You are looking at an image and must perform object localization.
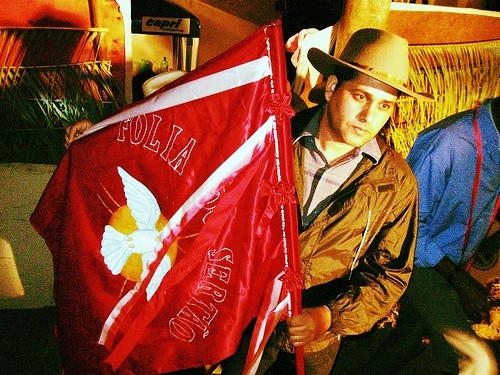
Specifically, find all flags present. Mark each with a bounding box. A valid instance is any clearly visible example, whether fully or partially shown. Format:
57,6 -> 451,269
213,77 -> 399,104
29,19 -> 304,375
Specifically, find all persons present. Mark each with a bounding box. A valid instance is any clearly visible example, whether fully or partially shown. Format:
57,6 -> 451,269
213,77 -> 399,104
65,28 -> 418,375
405,94 -> 500,375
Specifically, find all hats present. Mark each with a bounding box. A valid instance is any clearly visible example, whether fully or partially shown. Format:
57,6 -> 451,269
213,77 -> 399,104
307,28 -> 435,102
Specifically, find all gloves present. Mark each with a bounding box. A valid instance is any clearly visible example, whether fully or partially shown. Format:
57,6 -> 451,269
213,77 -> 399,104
435,256 -> 490,322
